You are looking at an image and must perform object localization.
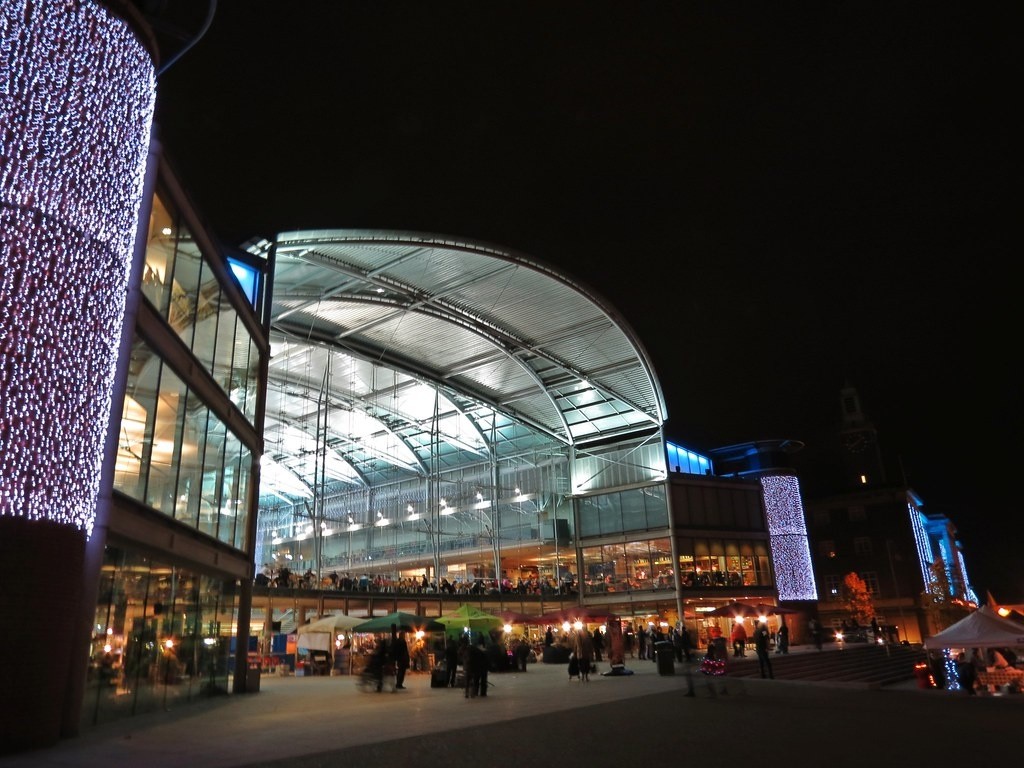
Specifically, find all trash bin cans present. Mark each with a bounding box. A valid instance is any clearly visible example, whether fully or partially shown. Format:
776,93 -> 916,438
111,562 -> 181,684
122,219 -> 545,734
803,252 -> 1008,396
655,641 -> 675,676
246,661 -> 262,693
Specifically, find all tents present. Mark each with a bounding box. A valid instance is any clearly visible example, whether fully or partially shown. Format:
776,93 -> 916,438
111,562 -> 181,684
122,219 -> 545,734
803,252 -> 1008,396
296,602 -> 620,636
925,604 -> 1024,649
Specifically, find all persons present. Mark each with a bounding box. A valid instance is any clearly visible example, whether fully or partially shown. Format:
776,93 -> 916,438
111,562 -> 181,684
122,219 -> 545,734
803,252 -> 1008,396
356,616 -> 860,699
870,616 -> 890,645
259,561 -> 748,598
929,647 -> 1017,697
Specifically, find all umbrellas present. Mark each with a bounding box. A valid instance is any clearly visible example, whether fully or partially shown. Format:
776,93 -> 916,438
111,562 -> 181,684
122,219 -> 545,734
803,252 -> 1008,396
703,603 -> 798,619
